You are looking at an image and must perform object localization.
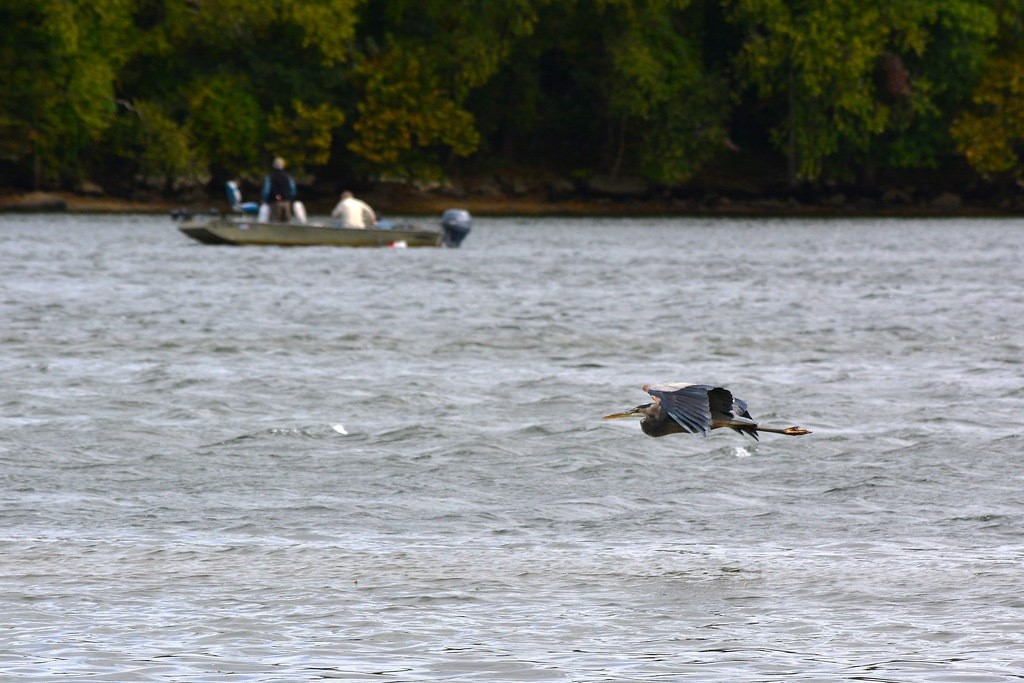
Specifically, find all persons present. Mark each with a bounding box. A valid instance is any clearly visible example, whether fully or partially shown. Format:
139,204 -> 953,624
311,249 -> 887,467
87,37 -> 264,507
261,156 -> 298,225
330,191 -> 377,229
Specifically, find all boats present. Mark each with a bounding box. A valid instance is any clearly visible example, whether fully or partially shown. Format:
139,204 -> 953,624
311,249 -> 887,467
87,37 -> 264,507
179,207 -> 474,249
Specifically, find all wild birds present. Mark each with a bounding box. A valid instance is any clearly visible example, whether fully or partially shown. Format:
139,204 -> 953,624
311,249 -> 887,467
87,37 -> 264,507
600,383 -> 813,442
